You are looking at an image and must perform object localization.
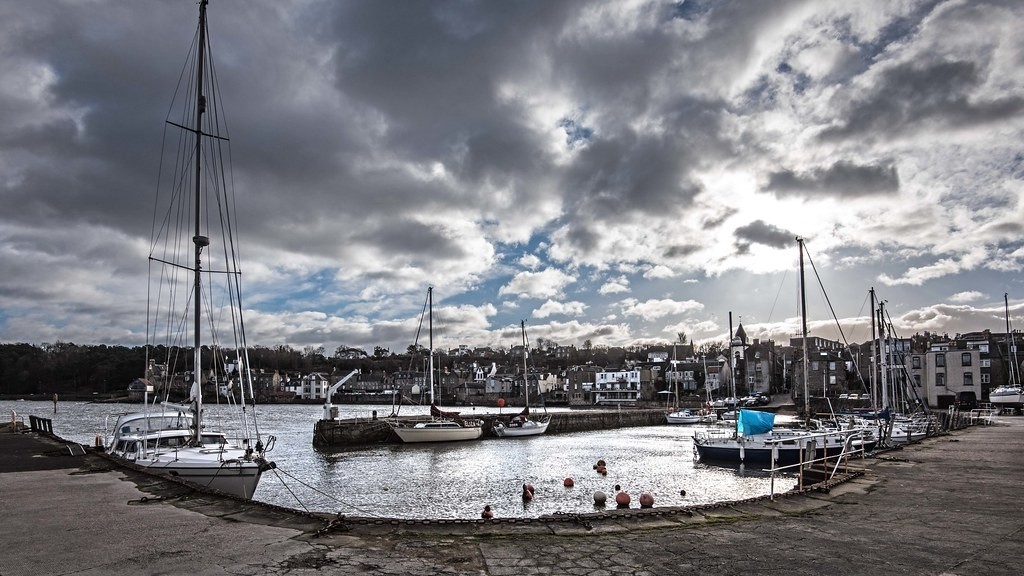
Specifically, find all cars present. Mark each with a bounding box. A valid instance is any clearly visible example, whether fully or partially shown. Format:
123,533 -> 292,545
849,395 -> 858,400
838,393 -> 848,399
860,393 -> 871,400
706,395 -> 771,407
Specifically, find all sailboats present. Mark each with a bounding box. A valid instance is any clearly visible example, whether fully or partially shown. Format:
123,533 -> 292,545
690,234 -> 877,470
989,292 -> 1024,414
492,320 -> 552,438
664,342 -> 701,423
770,287 -> 941,445
108,0 -> 264,501
384,284 -> 484,443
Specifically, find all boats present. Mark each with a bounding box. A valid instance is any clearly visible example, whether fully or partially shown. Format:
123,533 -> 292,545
17,399 -> 25,402
